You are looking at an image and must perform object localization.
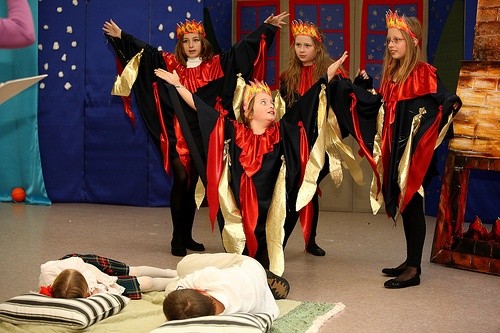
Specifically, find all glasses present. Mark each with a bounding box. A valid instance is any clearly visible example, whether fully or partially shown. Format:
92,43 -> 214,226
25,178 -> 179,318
385,36 -> 404,44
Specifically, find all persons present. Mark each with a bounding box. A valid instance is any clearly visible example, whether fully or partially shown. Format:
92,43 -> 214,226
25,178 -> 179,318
101,10 -> 290,257
153,49 -> 350,276
0,0 -> 35,49
345,8 -> 462,288
278,19 -> 373,257
38,253 -> 176,298
162,253 -> 290,320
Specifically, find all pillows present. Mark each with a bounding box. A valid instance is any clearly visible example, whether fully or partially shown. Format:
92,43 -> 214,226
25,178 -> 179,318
0,294 -> 131,329
149,313 -> 274,333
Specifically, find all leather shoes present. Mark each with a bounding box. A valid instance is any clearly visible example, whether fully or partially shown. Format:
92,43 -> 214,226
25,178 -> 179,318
382,267 -> 421,276
383,278 -> 421,288
190,239 -> 205,252
170,240 -> 188,257
306,245 -> 325,256
